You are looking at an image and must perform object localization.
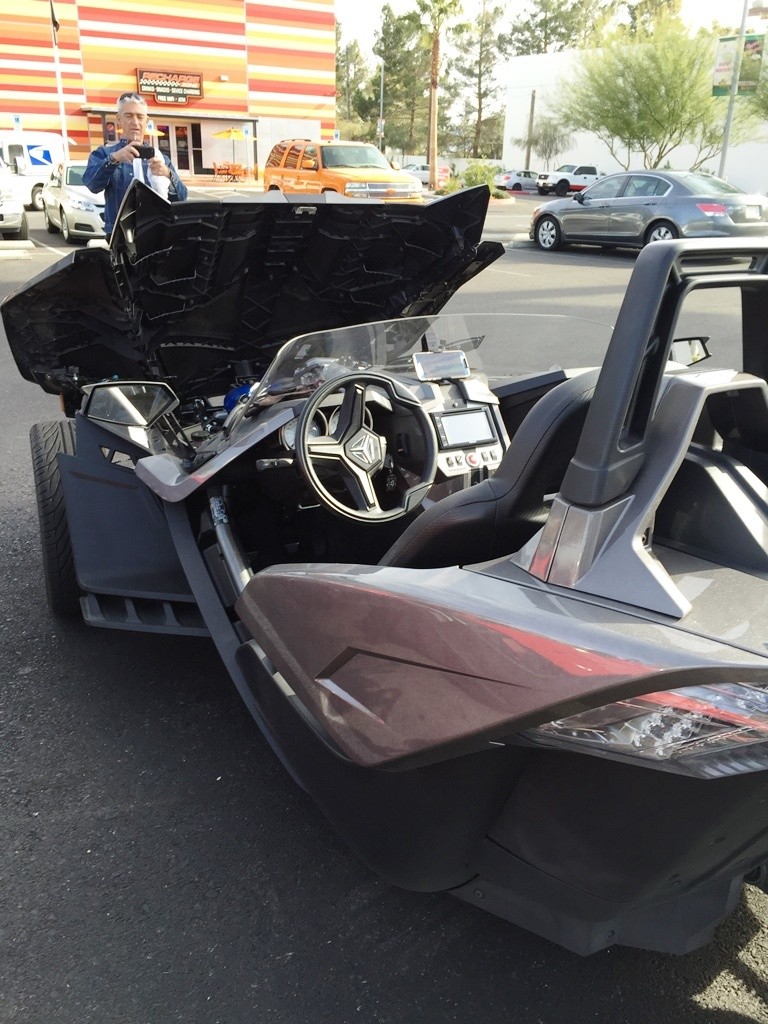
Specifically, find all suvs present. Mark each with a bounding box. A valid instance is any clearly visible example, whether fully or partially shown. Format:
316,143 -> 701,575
535,165 -> 608,196
264,138 -> 425,199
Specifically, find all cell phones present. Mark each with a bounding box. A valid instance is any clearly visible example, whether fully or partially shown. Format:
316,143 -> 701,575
412,350 -> 470,381
132,146 -> 154,158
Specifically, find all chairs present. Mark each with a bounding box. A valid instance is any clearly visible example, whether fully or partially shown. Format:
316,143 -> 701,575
300,368 -> 606,568
69,173 -> 82,184
633,187 -> 648,196
212,160 -> 248,183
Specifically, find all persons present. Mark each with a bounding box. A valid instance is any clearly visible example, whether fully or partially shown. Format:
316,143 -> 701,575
82,92 -> 187,246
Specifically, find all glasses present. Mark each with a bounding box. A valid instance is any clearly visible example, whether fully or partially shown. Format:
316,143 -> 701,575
119,93 -> 144,101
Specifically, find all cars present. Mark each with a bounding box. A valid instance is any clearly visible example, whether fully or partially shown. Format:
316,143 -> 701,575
529,168 -> 768,257
398,164 -> 431,185
493,170 -> 542,190
0,182 -> 28,240
41,158 -> 111,245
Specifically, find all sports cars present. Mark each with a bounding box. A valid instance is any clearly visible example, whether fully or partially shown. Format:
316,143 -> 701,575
1,181 -> 768,961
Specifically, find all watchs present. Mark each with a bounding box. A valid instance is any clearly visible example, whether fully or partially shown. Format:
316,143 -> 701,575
109,152 -> 118,163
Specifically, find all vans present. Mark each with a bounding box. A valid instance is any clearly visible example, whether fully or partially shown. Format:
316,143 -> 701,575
0,129 -> 77,211
